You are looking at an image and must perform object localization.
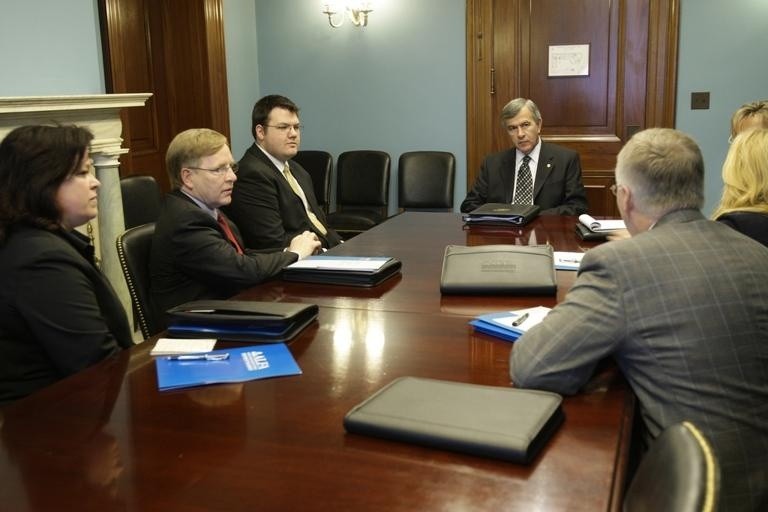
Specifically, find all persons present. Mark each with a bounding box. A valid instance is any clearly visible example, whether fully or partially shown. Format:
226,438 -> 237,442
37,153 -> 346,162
459,97 -> 589,214
232,96 -> 344,253
0,123 -> 134,404
149,129 -> 322,317
710,130 -> 768,248
729,102 -> 768,144
510,128 -> 768,512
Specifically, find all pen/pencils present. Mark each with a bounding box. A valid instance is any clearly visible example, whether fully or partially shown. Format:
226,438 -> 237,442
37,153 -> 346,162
564,259 -> 581,263
165,353 -> 230,361
513,313 -> 528,327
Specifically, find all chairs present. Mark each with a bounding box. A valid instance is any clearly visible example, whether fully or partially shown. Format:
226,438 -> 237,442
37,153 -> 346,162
289,150 -> 333,216
120,174 -> 161,230
115,220 -> 191,341
393,151 -> 455,210
324,150 -> 390,231
614,420 -> 722,511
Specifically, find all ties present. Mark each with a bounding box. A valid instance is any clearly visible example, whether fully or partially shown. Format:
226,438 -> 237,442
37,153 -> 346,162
216,212 -> 244,255
514,157 -> 533,207
283,165 -> 328,237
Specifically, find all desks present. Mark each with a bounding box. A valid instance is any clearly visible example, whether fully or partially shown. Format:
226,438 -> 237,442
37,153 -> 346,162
0,211 -> 640,511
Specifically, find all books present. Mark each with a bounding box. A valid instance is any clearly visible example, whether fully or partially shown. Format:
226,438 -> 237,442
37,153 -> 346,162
343,376 -> 562,462
286,254 -> 393,274
150,337 -> 217,356
283,259 -> 400,289
155,341 -> 305,394
473,304 -> 552,343
574,222 -> 611,245
577,213 -> 626,234
462,202 -> 539,226
167,299 -> 319,345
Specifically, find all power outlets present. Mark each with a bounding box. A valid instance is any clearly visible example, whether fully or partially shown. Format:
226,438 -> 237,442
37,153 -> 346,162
691,92 -> 710,109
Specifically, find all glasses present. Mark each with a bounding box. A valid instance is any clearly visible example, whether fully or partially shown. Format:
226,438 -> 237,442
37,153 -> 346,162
261,123 -> 303,133
183,163 -> 239,176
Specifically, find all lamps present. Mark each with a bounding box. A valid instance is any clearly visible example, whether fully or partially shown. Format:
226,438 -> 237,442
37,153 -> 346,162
320,0 -> 373,28
